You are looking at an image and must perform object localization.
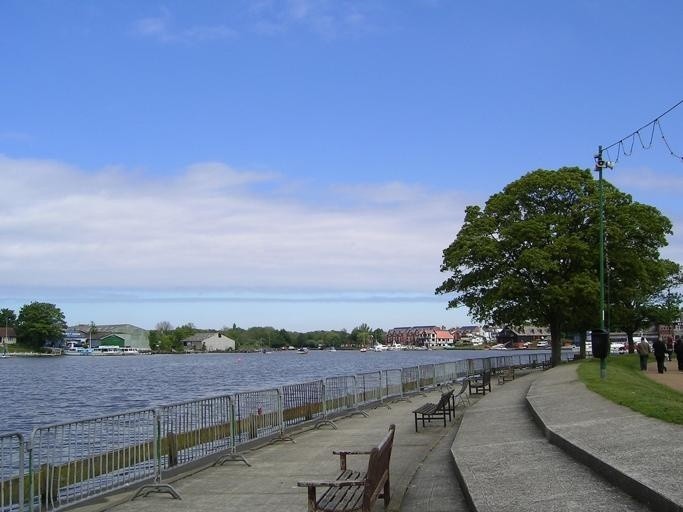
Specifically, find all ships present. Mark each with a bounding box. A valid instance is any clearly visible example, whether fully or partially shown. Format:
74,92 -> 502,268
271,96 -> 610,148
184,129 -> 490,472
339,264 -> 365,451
570,333 -> 652,355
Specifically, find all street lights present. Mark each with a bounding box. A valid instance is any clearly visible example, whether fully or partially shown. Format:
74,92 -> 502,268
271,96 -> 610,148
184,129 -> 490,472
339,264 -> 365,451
593,143 -> 613,377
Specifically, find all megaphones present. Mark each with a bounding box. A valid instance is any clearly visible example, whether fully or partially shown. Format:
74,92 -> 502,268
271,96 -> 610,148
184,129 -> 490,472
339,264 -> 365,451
597,159 -> 606,169
607,159 -> 617,171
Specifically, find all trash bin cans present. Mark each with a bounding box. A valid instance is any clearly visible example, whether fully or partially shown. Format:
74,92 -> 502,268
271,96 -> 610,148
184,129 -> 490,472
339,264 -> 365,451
591,331 -> 609,359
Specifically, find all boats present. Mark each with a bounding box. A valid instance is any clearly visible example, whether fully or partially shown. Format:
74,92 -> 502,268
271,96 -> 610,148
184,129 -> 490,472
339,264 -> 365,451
523,341 -> 532,347
359,347 -> 366,352
536,341 -> 548,347
119,346 -> 139,355
367,339 -> 432,353
90,344 -> 123,356
280,344 -> 336,354
487,343 -> 506,351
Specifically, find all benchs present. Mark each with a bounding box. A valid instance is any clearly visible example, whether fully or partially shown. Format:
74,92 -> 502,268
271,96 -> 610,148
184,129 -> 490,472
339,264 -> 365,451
470,354 -> 580,395
414,389 -> 456,432
296,423 -> 396,512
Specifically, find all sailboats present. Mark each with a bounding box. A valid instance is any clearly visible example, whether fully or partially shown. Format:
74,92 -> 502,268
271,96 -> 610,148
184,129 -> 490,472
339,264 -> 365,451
0,318 -> 15,359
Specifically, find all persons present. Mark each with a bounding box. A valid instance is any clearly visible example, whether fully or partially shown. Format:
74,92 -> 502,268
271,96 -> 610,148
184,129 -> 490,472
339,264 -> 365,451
636,336 -> 650,371
673,334 -> 683,370
651,333 -> 668,374
665,337 -> 674,361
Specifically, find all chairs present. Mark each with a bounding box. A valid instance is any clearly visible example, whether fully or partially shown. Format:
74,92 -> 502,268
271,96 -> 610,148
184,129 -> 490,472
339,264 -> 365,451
454,379 -> 470,406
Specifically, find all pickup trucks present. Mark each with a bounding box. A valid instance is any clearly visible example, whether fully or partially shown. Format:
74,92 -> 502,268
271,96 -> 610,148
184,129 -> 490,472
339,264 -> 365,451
61,341 -> 92,357
